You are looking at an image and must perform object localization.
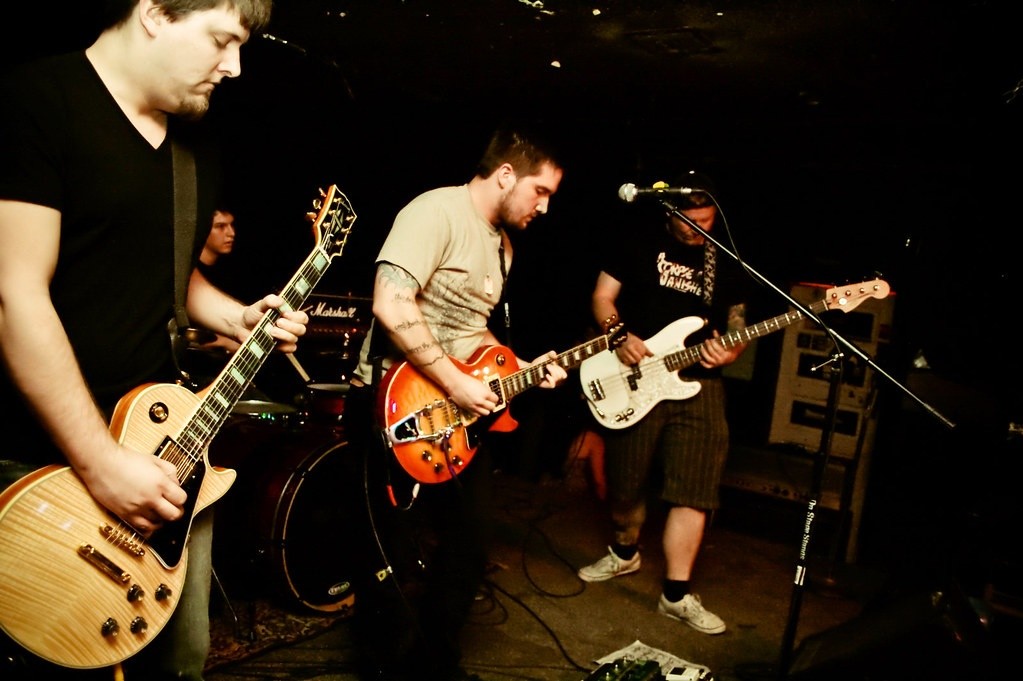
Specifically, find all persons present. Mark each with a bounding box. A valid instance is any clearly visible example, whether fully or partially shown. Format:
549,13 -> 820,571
579,188 -> 747,634
0,0 -> 310,680
352,131 -> 569,681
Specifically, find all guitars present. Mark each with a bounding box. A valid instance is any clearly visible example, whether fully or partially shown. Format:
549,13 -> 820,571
578,270 -> 892,432
373,310 -> 631,487
0,181 -> 357,671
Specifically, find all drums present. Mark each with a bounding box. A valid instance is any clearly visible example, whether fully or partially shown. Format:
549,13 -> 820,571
301,381 -> 352,437
210,430 -> 389,615
218,400 -> 299,450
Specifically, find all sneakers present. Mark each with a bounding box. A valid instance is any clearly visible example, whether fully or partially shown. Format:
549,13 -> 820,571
576,543 -> 643,583
655,591 -> 727,635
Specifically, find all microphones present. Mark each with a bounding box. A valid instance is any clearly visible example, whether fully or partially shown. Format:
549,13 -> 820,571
618,182 -> 692,202
